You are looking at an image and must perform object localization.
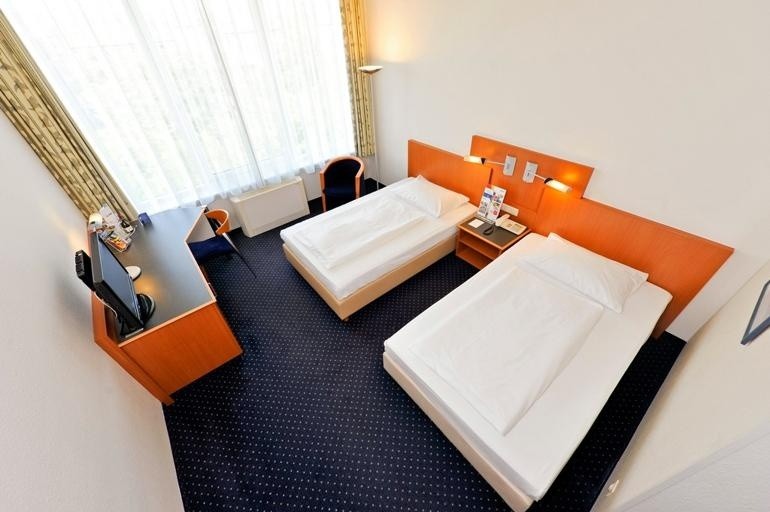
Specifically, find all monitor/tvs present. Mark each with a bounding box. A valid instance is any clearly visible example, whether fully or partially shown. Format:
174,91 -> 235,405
89,231 -> 155,338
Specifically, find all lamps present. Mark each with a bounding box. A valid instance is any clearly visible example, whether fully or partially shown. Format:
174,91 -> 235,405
464,154 -> 517,176
359,66 -> 384,190
521,160 -> 573,194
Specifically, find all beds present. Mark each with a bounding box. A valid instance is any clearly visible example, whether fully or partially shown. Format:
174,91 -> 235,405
381,235 -> 673,512
280,176 -> 480,321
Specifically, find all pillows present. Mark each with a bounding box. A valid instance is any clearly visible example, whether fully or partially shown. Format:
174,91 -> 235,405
390,173 -> 471,218
525,231 -> 649,315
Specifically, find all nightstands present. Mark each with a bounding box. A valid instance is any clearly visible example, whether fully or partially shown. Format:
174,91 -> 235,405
456,210 -> 533,270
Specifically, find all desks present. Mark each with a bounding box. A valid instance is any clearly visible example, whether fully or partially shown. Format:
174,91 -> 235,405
87,205 -> 244,407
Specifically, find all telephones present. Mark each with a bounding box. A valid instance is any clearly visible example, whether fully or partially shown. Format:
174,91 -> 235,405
495,214 -> 527,236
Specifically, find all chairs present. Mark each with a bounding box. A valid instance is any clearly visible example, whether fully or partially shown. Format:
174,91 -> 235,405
187,209 -> 257,279
320,156 -> 365,212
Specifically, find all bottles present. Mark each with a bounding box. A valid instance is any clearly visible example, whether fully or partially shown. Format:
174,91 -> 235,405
121,219 -> 133,234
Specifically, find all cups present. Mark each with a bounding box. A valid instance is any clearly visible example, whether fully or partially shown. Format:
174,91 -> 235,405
138,212 -> 151,226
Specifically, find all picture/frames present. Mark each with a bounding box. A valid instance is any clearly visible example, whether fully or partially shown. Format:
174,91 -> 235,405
740,279 -> 770,345
